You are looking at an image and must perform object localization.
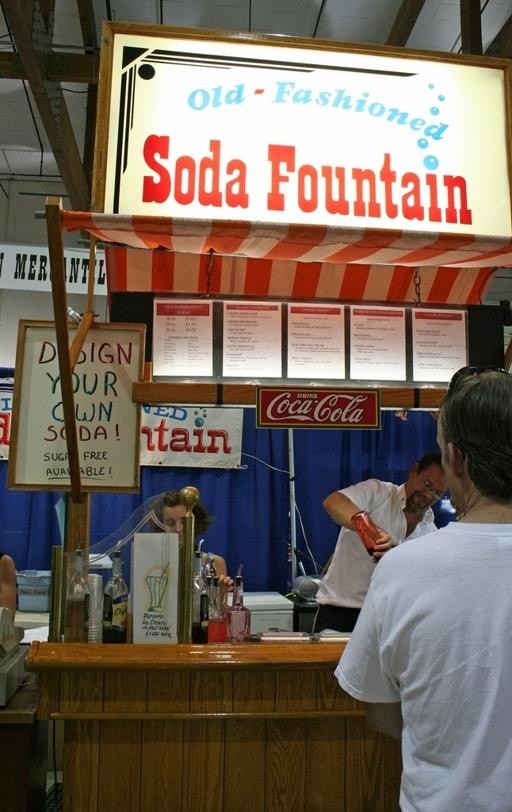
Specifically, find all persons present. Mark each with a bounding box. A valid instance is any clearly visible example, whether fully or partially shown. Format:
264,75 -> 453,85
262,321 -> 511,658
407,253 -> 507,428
310,450 -> 447,634
334,367 -> 512,812
155,489 -> 234,607
0,551 -> 18,626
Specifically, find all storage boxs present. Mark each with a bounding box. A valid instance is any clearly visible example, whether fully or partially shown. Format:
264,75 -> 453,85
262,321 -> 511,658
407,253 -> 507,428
15,570 -> 52,612
88,553 -> 113,588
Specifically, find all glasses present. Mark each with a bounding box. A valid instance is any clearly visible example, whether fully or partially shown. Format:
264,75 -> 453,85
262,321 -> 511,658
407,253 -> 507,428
447,366 -> 509,391
420,467 -> 447,502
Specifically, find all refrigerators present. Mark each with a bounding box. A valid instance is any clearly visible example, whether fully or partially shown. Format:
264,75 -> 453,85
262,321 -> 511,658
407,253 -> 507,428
224,589 -> 295,637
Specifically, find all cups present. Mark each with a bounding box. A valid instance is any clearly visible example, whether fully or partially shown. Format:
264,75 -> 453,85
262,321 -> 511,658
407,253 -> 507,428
85,571 -> 105,644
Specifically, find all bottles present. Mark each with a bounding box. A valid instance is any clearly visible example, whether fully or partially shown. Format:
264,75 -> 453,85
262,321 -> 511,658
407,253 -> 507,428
350,511 -> 384,564
102,550 -> 130,645
190,548 -> 254,646
64,548 -> 89,644
